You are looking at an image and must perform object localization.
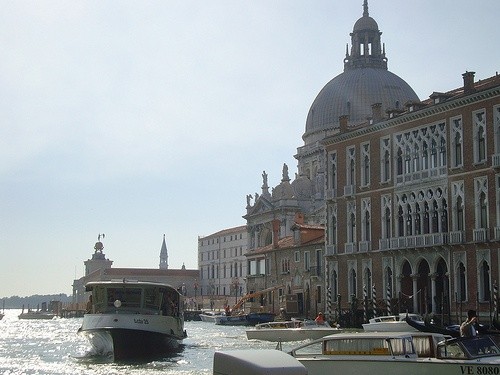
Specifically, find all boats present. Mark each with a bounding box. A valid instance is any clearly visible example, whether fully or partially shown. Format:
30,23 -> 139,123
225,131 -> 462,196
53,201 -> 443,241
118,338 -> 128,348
18,313 -> 57,319
199,312 -> 276,325
287,331 -> 500,375
246,322 -> 343,342
77,280 -> 188,363
361,316 -> 434,332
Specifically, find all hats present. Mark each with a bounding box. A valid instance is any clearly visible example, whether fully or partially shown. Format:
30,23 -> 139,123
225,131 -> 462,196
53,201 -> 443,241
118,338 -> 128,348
468,310 -> 478,317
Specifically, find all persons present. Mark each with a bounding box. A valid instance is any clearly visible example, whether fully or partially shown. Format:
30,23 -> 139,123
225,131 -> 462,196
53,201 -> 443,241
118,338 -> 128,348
86,295 -> 92,310
460,310 -> 483,337
163,298 -> 170,315
226,305 -> 230,316
316,312 -> 323,325
